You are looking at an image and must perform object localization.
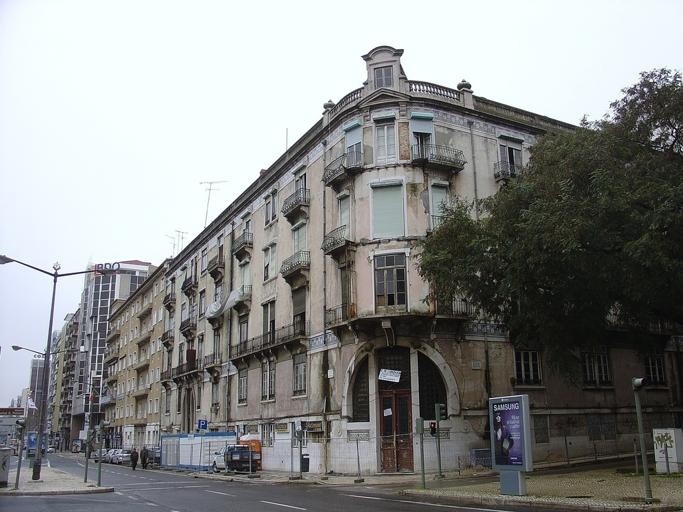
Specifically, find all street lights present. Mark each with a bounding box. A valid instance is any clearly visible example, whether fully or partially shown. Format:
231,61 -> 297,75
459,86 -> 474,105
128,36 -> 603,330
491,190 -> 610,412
10,343 -> 79,468
0,251 -> 116,485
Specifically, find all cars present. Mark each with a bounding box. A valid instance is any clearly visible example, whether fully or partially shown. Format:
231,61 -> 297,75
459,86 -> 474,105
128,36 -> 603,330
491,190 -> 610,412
21,430 -> 160,472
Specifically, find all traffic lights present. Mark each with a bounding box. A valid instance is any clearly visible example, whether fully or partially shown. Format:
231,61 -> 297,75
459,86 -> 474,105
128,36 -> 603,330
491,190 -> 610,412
434,403 -> 446,423
16,418 -> 25,427
429,421 -> 436,437
631,377 -> 648,390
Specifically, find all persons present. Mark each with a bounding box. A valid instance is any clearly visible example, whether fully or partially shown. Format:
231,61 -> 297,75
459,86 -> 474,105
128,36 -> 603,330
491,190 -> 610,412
129,448 -> 138,470
140,446 -> 148,468
494,412 -> 514,464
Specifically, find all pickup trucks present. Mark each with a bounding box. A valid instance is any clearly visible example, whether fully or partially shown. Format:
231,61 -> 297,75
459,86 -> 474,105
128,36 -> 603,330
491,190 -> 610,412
210,443 -> 261,475
